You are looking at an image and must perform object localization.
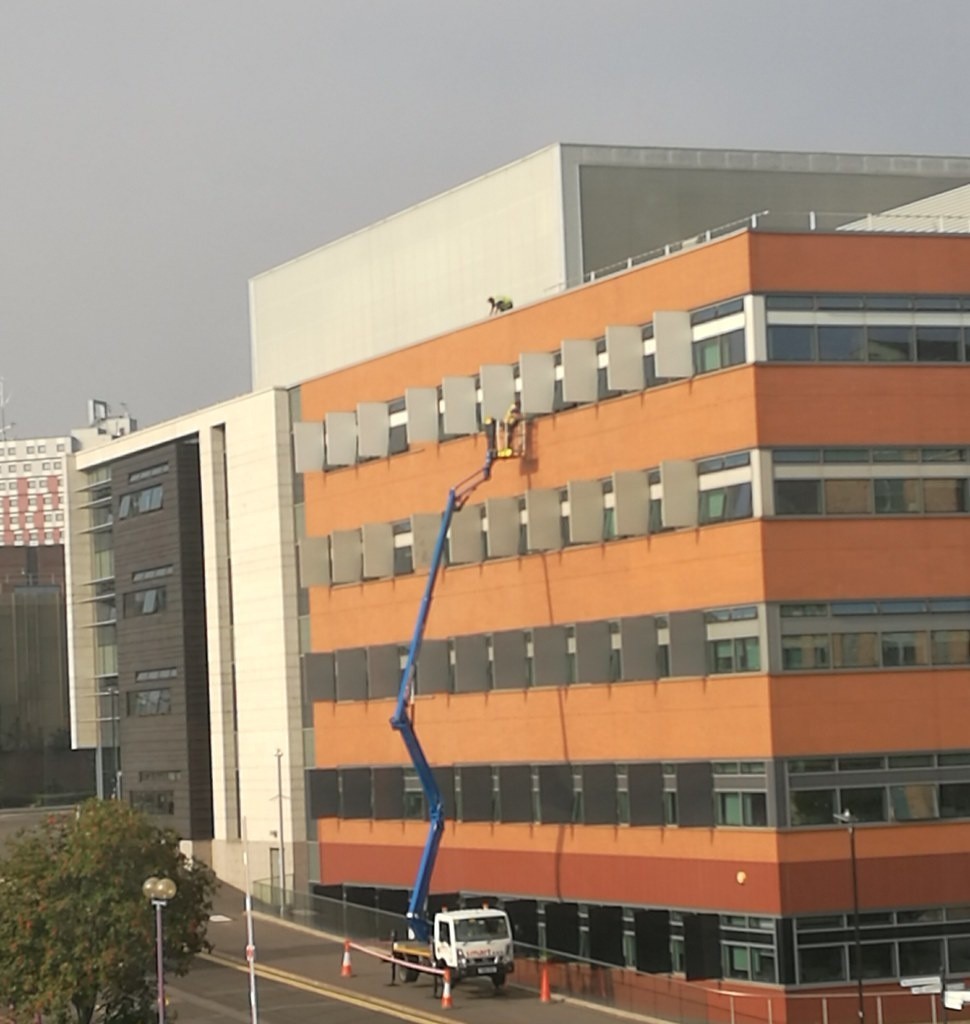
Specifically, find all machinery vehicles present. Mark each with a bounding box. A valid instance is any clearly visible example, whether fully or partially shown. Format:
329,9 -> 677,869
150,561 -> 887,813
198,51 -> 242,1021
387,406 -> 523,992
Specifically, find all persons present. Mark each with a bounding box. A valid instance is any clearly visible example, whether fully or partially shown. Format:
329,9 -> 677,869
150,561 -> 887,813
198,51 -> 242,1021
489,296 -> 514,316
501,400 -> 523,455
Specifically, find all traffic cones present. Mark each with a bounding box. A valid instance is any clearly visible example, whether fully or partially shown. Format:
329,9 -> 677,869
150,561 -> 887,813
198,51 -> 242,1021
339,939 -> 354,981
540,969 -> 550,1004
437,966 -> 457,1011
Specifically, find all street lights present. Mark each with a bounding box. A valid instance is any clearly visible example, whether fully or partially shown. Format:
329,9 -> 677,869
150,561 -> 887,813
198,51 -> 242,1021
106,684 -> 118,802
141,875 -> 179,1024
272,745 -> 289,919
834,806 -> 865,1023
